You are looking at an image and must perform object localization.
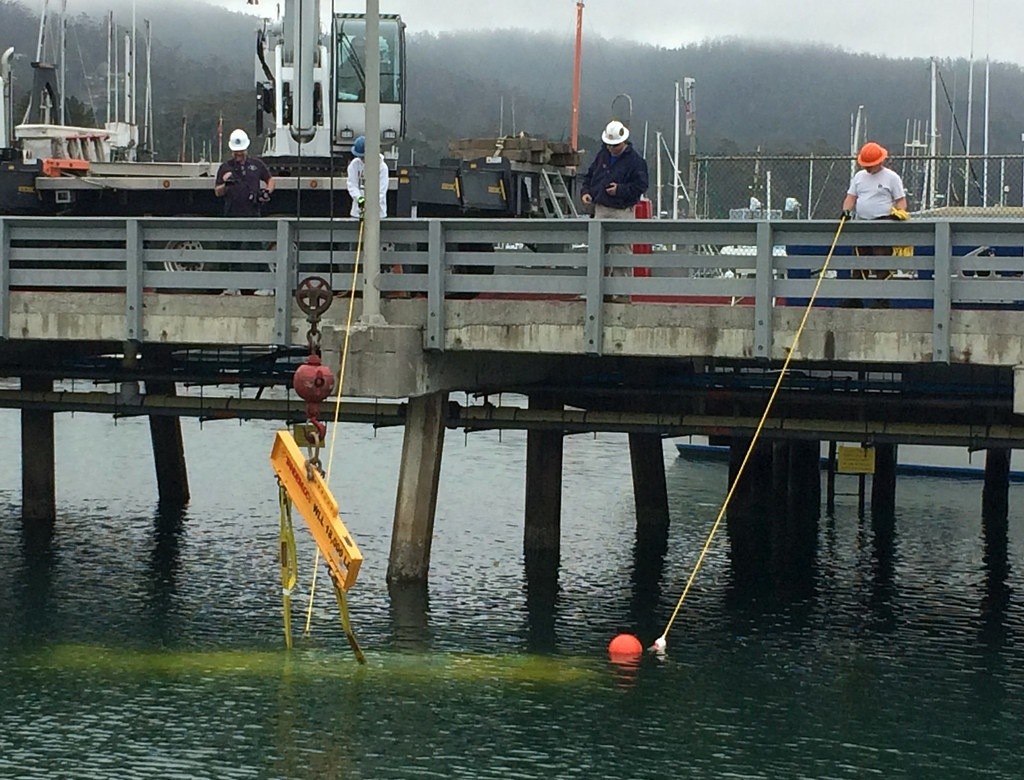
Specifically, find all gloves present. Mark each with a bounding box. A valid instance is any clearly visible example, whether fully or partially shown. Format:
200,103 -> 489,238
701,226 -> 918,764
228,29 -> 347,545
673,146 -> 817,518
357,196 -> 367,209
840,210 -> 852,221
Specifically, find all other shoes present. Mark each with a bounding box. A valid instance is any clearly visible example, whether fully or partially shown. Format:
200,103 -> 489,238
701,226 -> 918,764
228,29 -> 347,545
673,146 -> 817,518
609,294 -> 632,304
870,298 -> 891,308
843,298 -> 864,308
577,294 -> 586,301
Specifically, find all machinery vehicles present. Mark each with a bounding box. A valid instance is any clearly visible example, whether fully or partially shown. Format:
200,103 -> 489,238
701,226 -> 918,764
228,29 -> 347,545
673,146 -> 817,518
0,0 -> 587,593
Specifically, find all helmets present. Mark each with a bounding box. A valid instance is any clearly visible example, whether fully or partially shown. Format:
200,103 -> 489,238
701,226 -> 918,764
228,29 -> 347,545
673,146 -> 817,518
351,135 -> 366,156
858,142 -> 888,166
601,120 -> 630,144
228,129 -> 250,151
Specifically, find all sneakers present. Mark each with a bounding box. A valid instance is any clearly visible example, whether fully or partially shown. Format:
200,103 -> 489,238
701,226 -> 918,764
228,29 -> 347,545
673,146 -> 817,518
254,288 -> 275,296
222,288 -> 242,296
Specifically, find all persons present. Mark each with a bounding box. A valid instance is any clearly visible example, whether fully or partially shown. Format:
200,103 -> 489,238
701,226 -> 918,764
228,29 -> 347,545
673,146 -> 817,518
335,35 -> 367,97
215,129 -> 276,297
346,136 -> 389,297
839,142 -> 907,309
565,120 -> 649,306
